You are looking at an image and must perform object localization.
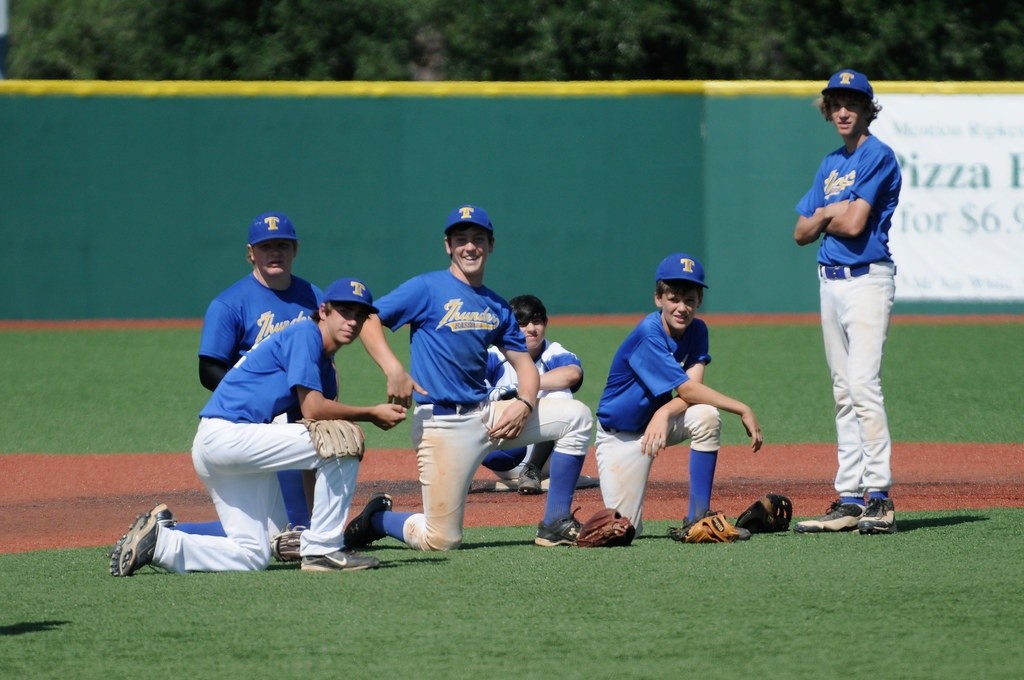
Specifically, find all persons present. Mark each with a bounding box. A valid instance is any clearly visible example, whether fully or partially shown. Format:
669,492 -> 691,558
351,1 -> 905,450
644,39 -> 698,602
794,70 -> 902,535
468,295 -> 600,496
595,253 -> 763,540
342,205 -> 594,551
110,278 -> 408,571
149,214 -> 339,538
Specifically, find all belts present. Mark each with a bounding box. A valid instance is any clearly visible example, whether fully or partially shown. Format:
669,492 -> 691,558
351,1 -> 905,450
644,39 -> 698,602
601,423 -> 620,433
820,263 -> 869,279
433,402 -> 480,416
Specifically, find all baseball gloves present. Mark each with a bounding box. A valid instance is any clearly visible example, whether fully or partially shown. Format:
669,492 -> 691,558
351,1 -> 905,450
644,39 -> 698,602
294,415 -> 367,462
576,508 -> 636,547
684,514 -> 739,544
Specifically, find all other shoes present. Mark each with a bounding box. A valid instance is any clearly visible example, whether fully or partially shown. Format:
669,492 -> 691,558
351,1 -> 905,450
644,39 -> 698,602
737,527 -> 752,540
148,503 -> 177,528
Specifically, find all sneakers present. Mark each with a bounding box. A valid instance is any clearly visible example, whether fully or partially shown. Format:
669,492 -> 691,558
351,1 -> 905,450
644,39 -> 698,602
534,506 -> 584,546
857,496 -> 897,534
793,499 -> 867,534
517,461 -> 542,495
301,551 -> 381,572
109,513 -> 162,577
343,493 -> 392,550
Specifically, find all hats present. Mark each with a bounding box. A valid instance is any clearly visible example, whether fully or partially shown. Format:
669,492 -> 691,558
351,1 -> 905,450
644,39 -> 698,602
249,212 -> 302,245
821,69 -> 874,100
655,253 -> 709,289
321,278 -> 380,314
444,204 -> 493,234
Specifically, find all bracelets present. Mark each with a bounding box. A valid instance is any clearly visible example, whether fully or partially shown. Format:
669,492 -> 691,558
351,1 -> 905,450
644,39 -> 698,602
518,398 -> 533,412
